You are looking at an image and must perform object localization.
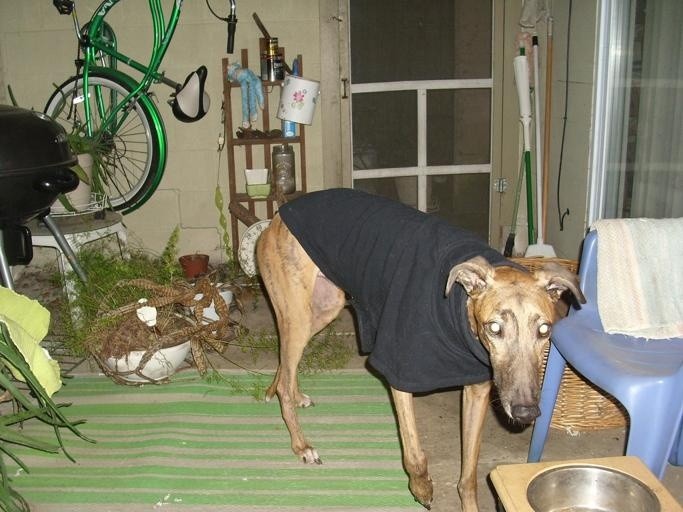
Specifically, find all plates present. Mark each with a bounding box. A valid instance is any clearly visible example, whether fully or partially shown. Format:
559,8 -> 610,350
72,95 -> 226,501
238,220 -> 273,279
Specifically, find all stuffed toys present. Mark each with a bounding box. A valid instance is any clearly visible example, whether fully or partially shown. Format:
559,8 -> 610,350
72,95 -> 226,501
227,62 -> 264,128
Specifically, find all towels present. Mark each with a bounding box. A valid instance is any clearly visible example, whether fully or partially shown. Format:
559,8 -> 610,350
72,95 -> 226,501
590,217 -> 683,340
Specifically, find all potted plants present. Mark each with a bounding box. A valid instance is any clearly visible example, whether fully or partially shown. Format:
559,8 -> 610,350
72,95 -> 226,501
52,226 -> 354,403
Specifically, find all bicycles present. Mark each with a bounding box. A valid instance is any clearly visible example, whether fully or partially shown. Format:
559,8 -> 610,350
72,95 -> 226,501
43,0 -> 238,216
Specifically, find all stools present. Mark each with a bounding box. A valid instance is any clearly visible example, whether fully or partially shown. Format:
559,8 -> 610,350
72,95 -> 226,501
8,212 -> 132,338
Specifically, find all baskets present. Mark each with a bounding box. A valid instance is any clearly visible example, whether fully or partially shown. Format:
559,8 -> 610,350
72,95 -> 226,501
511,258 -> 632,434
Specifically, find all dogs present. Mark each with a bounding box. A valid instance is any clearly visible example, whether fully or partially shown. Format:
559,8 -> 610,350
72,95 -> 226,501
256,188 -> 586,512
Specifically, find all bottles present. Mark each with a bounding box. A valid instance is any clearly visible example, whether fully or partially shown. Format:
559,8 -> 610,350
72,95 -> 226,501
273,144 -> 295,195
281,121 -> 295,136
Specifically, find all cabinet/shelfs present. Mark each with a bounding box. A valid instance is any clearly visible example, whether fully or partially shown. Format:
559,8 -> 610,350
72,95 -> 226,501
221,49 -> 307,277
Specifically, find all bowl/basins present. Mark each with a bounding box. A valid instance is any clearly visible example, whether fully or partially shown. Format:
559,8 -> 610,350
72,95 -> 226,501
526,466 -> 660,512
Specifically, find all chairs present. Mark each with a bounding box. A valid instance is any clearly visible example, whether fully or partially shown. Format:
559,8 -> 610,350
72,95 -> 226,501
525,218 -> 683,481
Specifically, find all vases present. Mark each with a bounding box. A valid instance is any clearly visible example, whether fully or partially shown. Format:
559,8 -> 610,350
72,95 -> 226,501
178,254 -> 209,279
182,283 -> 233,337
